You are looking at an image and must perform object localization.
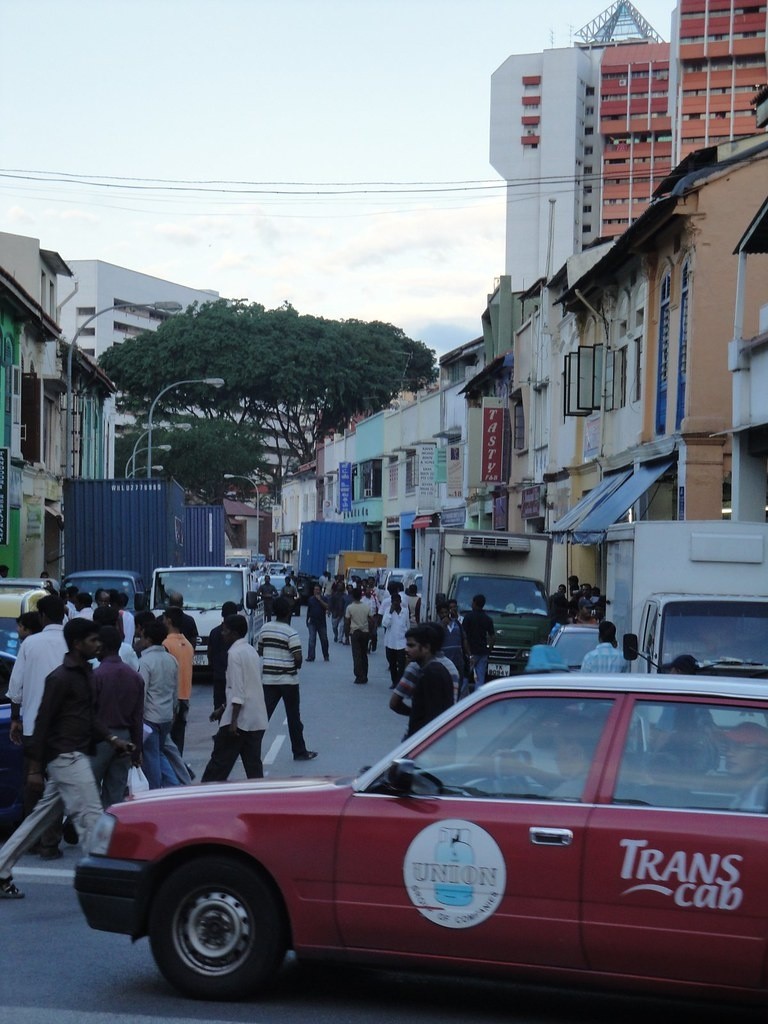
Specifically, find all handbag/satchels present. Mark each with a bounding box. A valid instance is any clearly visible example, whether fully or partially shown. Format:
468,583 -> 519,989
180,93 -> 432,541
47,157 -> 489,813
124,765 -> 150,796
463,656 -> 476,683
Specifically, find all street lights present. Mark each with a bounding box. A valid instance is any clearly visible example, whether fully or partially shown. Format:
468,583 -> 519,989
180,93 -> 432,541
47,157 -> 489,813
66,299 -> 183,478
224,474 -> 259,554
148,378 -> 226,477
132,423 -> 192,476
125,445 -> 171,478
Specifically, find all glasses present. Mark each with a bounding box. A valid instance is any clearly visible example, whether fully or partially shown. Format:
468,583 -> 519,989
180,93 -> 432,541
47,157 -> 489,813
725,743 -> 763,752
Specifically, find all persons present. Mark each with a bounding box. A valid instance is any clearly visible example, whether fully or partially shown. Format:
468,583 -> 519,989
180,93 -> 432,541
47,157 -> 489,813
481,725 -> 598,801
305,583 -> 330,663
345,588 -> 372,686
0,618 -> 138,899
654,655 -> 713,726
200,613 -> 269,783
0,562 -> 633,855
257,596 -> 318,761
713,722 -> 768,811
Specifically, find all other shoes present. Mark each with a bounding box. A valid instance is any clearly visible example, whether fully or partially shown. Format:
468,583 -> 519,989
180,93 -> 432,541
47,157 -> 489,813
324,655 -> 329,661
294,750 -> 318,760
0,875 -> 25,899
305,658 -> 314,662
62,818 -> 79,845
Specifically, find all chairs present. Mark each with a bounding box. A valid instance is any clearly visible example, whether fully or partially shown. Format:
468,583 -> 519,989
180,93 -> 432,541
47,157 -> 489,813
620,742 -> 689,806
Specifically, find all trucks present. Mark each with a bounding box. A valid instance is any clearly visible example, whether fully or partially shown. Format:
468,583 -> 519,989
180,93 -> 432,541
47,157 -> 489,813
605,520 -> 768,680
337,550 -> 388,590
418,526 -> 553,681
148,565 -> 267,676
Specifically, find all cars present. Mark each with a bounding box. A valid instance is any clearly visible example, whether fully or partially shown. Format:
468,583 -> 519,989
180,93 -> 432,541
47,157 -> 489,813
0,650 -> 52,832
74,673 -> 768,1024
225,557 -> 259,568
260,562 -> 294,575
259,575 -> 300,615
550,623 -> 604,673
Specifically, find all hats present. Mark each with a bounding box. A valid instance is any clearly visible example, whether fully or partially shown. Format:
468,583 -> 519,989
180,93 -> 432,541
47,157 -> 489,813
721,721 -> 768,748
579,599 -> 593,607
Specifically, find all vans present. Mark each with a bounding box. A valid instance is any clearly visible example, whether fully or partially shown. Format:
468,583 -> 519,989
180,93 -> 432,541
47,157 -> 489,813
373,568 -> 414,605
0,576 -> 61,595
0,586 -> 52,659
59,569 -> 147,616
403,571 -> 423,599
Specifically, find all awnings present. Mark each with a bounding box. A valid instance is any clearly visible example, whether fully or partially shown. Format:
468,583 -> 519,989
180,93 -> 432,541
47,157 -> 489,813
547,463 -> 676,540
224,497 -> 273,519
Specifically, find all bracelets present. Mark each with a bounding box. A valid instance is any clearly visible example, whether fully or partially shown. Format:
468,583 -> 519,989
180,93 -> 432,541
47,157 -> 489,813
222,703 -> 226,709
109,736 -> 118,742
